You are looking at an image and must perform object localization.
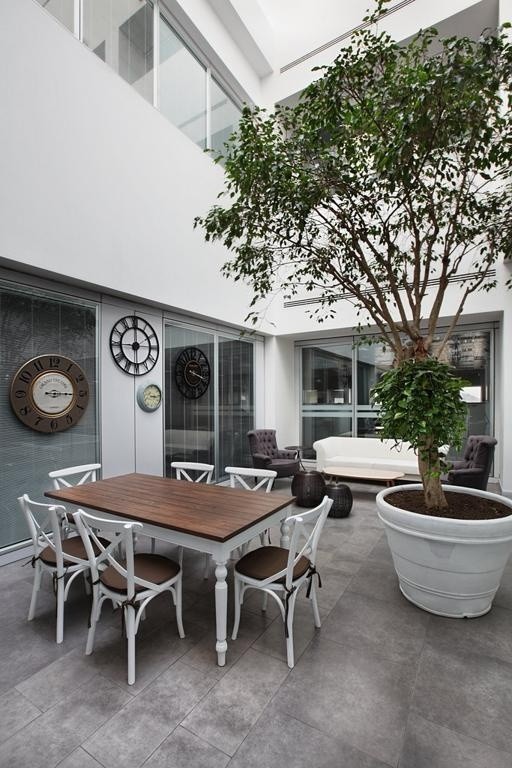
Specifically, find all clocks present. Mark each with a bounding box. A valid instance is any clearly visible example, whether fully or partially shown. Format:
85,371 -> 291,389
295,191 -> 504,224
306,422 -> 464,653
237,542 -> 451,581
10,315 -> 211,436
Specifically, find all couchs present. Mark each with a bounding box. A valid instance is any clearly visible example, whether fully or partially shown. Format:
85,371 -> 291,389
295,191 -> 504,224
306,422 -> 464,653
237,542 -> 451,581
312,436 -> 450,482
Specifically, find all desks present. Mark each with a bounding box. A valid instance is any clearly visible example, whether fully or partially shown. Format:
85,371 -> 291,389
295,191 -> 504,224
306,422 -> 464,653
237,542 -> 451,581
283,446 -> 313,471
43,473 -> 297,667
322,466 -> 406,488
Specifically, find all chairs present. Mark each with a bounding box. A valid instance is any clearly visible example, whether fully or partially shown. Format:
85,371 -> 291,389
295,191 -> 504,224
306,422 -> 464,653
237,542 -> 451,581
17,493 -> 119,644
47,460 -> 122,540
246,429 -> 300,485
445,435 -> 498,491
72,507 -> 187,685
152,461 -> 215,573
204,466 -> 280,578
231,495 -> 335,669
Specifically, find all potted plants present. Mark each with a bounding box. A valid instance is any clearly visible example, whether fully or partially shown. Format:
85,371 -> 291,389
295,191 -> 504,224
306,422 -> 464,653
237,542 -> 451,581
193,0 -> 512,618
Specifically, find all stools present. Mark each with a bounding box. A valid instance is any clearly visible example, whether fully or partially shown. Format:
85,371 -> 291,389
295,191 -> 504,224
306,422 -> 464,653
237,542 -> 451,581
322,484 -> 354,519
290,470 -> 326,507
